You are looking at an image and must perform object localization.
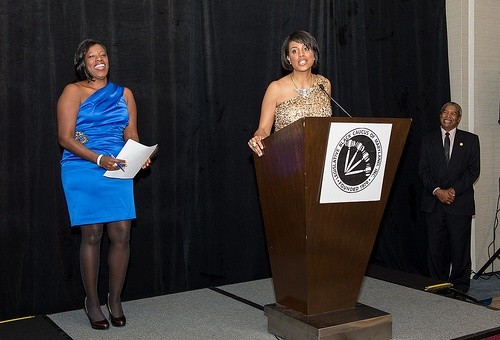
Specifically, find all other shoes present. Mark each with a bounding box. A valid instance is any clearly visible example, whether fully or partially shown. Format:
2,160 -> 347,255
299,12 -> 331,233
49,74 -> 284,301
453,291 -> 466,301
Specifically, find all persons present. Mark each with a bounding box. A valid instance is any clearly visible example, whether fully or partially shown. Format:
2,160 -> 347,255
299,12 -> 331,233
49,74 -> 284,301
57,39 -> 151,330
248,31 -> 332,157
417,102 -> 480,300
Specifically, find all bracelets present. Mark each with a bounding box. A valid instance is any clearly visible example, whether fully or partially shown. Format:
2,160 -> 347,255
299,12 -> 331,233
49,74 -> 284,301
96,154 -> 103,166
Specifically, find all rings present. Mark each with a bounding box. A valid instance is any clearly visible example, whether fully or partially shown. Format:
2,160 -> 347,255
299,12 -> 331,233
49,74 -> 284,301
253,142 -> 256,147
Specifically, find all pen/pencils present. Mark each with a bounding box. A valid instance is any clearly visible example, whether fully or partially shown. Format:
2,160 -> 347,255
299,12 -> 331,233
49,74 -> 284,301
106,151 -> 126,173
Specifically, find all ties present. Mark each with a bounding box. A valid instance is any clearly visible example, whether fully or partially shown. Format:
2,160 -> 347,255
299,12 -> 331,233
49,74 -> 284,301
442,131 -> 451,160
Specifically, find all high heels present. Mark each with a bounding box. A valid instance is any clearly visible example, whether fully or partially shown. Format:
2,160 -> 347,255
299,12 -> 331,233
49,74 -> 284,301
102,296 -> 127,329
82,294 -> 109,333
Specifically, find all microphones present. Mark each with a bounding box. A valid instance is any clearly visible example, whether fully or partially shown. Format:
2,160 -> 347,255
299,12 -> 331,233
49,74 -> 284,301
319,84 -> 352,117
